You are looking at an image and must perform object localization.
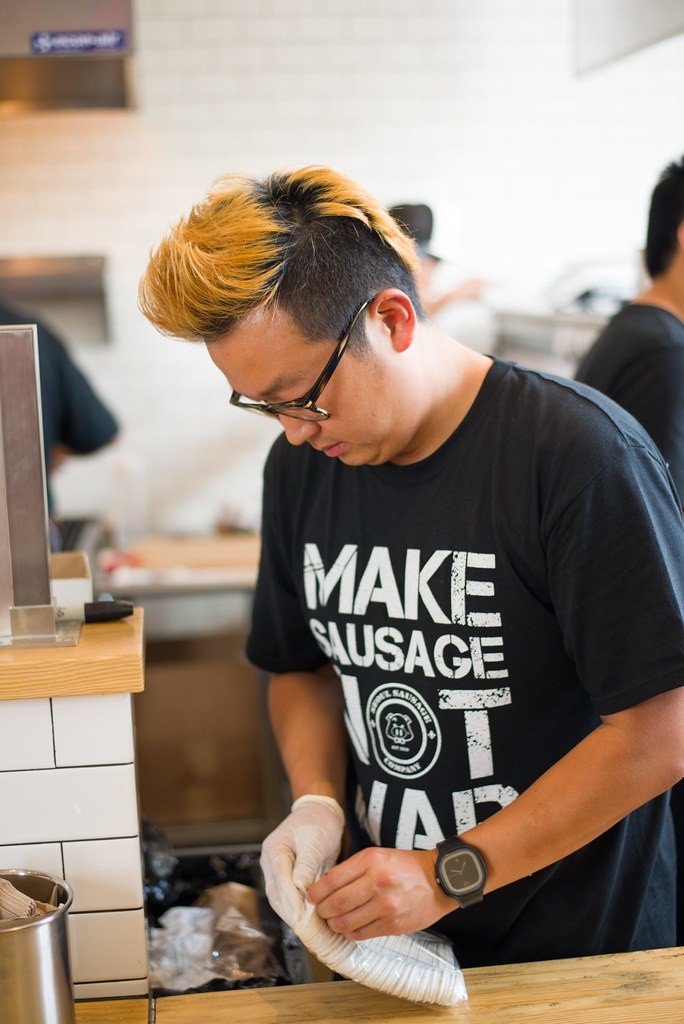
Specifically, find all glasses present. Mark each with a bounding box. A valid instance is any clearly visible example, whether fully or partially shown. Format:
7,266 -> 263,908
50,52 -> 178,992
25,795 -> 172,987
229,294 -> 376,420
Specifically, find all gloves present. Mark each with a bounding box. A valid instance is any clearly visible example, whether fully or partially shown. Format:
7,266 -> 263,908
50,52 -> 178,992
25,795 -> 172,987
261,795 -> 346,928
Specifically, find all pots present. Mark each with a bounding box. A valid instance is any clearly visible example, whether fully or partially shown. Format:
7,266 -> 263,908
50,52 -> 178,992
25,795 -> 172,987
0,867 -> 76,1024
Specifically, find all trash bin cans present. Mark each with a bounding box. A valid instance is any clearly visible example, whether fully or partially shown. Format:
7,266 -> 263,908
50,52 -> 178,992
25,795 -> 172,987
147,841 -> 316,997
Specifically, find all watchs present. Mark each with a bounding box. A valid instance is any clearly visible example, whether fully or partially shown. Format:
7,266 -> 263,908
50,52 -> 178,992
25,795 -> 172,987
435,835 -> 486,910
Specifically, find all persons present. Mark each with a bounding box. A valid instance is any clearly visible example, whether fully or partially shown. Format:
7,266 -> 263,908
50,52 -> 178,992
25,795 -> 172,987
576,156 -> 684,511
386,203 -> 498,355
0,295 -> 117,552
141,166 -> 684,970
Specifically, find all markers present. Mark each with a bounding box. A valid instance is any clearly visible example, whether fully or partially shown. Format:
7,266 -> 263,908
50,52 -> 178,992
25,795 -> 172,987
55,600 -> 136,629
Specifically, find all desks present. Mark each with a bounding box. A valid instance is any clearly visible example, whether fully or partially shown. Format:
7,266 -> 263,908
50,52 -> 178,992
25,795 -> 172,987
72,946 -> 684,1024
0,604 -> 145,700
